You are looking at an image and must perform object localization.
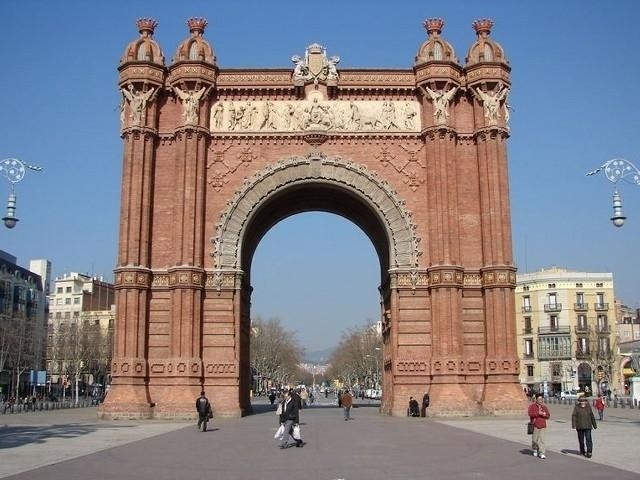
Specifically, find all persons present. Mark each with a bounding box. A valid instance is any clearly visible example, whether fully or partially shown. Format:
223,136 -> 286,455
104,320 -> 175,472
196,391 -> 209,432
595,394 -> 605,420
340,390 -> 352,421
337,392 -> 342,407
421,392 -> 429,417
213,101 -> 417,131
572,395 -> 597,458
409,397 -> 420,417
324,389 -> 328,398
528,393 -> 550,458
269,385 -> 314,448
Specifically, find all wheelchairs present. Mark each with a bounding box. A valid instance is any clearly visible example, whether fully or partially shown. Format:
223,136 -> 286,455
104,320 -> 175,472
410,406 -> 420,417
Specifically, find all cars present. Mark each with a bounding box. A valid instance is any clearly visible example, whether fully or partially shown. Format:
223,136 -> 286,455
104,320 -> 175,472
364,389 -> 382,399
561,389 -> 585,400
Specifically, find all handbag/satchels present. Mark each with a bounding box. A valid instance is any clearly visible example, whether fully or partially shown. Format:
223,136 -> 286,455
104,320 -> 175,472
276,403 -> 283,415
527,423 -> 534,434
293,424 -> 301,440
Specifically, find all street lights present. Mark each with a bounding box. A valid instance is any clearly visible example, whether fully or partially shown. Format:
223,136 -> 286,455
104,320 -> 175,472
366,355 -> 379,389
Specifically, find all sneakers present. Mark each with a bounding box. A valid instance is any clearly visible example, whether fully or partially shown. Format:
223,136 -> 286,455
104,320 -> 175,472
533,450 -> 538,456
539,453 -> 546,459
587,453 -> 592,457
